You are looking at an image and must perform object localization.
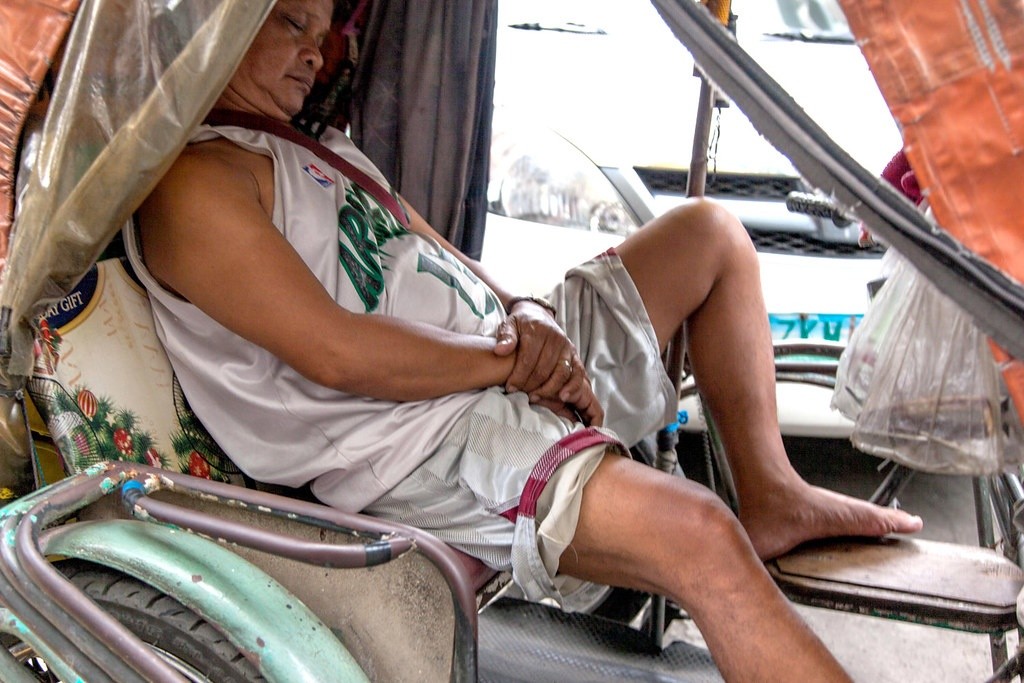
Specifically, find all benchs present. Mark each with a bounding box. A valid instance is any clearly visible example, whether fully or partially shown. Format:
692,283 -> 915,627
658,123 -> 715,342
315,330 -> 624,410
30,251 -> 516,614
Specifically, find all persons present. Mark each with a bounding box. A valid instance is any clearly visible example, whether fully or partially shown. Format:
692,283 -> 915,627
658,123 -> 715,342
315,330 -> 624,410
121,1 -> 923,683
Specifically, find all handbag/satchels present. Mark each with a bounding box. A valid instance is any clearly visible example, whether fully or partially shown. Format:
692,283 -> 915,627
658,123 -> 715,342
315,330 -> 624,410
830,207 -> 1000,476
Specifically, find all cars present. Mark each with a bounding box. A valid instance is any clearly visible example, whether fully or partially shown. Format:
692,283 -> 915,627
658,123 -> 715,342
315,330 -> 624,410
463,0 -> 936,438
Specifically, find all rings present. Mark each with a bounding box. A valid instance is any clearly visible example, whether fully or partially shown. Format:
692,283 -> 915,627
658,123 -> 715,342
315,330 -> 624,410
559,359 -> 572,370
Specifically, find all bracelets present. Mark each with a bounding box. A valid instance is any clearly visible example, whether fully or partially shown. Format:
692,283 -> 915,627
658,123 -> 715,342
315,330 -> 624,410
507,295 -> 558,318
